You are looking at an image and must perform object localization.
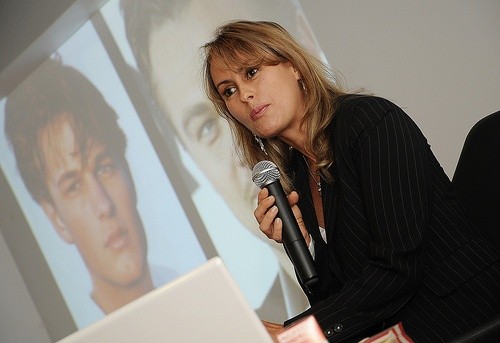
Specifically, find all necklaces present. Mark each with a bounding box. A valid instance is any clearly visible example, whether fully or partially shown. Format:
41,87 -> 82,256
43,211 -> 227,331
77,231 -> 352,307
302,154 -> 322,199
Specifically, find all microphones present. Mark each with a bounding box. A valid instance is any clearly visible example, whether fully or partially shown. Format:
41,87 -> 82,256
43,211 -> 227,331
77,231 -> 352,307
251,159 -> 324,293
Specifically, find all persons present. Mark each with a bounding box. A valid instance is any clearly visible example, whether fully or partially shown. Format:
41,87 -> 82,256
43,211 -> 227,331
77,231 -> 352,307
117,0 -> 335,324
4,55 -> 182,327
200,18 -> 500,343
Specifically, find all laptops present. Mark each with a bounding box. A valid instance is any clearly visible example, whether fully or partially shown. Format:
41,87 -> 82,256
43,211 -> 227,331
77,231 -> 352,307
59,259 -> 274,343
